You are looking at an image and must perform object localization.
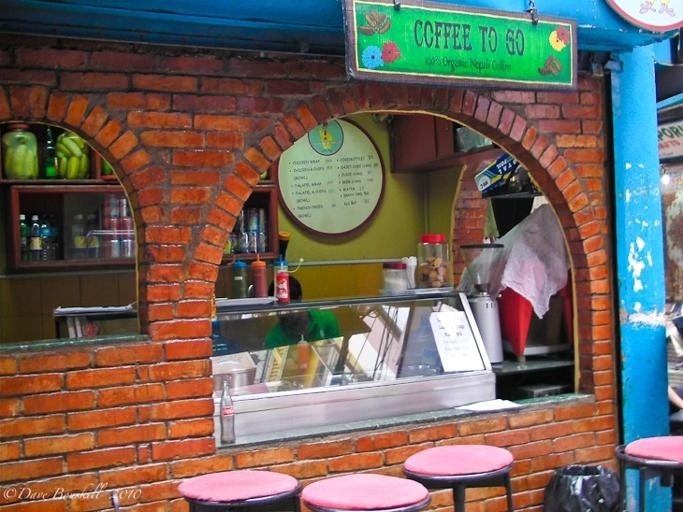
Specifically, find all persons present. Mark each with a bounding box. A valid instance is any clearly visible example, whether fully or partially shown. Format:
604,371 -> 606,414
262,275 -> 340,349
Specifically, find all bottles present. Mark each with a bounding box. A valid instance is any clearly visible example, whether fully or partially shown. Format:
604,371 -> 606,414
381,260 -> 408,292
232,256 -> 249,299
217,379 -> 238,447
70,212 -> 101,261
18,213 -> 58,261
250,254 -> 269,297
40,125 -> 56,178
272,255 -> 292,303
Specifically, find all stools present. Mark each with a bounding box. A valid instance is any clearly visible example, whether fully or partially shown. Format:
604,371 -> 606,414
301,472 -> 431,512
402,445 -> 513,511
178,470 -> 303,512
615,435 -> 683,512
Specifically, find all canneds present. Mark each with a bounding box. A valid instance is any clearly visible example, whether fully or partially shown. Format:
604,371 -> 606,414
2,123 -> 39,179
99,192 -> 134,259
56,130 -> 89,179
234,207 -> 268,253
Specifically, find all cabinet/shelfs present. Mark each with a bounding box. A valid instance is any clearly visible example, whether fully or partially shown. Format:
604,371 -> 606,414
52,291 -> 497,448
389,114 -> 503,174
2,124 -> 279,272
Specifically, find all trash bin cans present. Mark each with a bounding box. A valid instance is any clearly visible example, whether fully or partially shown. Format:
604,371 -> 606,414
554,464 -> 612,511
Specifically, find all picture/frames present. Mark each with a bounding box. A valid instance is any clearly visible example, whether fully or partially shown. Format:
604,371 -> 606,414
277,117 -> 385,238
